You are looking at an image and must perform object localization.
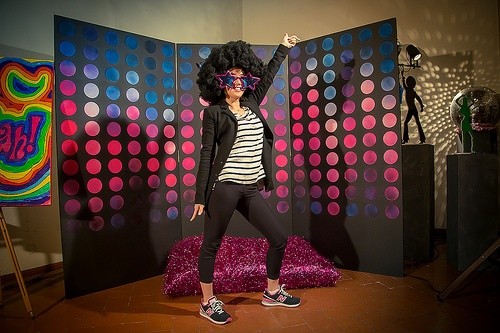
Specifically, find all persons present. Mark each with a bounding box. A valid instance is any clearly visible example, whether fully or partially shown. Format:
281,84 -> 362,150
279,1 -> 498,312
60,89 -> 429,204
189,33 -> 301,324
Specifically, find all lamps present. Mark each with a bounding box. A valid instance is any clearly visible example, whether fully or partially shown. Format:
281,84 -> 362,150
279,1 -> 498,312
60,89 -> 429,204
403,44 -> 422,68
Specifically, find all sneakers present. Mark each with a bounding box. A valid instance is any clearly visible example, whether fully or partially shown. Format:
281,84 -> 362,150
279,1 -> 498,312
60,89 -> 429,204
262,283 -> 301,307
198,296 -> 232,325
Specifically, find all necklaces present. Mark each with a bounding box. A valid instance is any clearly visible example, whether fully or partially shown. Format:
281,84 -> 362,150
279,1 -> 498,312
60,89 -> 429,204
236,107 -> 240,111
